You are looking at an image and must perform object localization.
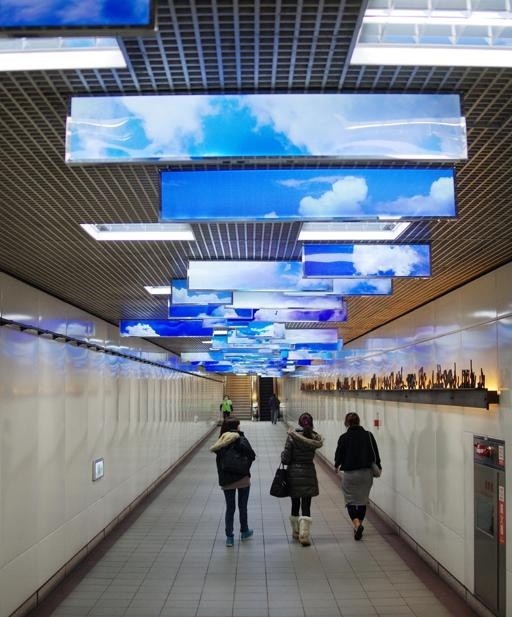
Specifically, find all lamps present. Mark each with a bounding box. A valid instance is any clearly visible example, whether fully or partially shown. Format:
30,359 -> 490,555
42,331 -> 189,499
202,327 -> 296,378
2,35 -> 130,71
80,222 -> 198,242
144,285 -> 173,296
349,0 -> 512,69
296,221 -> 413,242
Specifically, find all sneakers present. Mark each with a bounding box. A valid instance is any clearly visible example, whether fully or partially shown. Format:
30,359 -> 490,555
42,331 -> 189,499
241,530 -> 253,539
226,536 -> 234,546
354,525 -> 363,540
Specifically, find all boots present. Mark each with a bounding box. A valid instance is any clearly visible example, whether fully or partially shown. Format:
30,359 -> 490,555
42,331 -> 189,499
298,516 -> 311,546
289,515 -> 299,538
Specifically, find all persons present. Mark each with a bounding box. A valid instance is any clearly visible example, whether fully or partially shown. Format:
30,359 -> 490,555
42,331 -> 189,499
281,412 -> 325,546
220,395 -> 233,419
334,412 -> 382,540
251,399 -> 260,421
269,393 -> 280,424
299,369 -> 485,389
209,417 -> 254,546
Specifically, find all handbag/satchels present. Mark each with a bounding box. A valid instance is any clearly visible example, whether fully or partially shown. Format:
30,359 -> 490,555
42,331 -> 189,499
270,468 -> 290,497
373,463 -> 380,477
